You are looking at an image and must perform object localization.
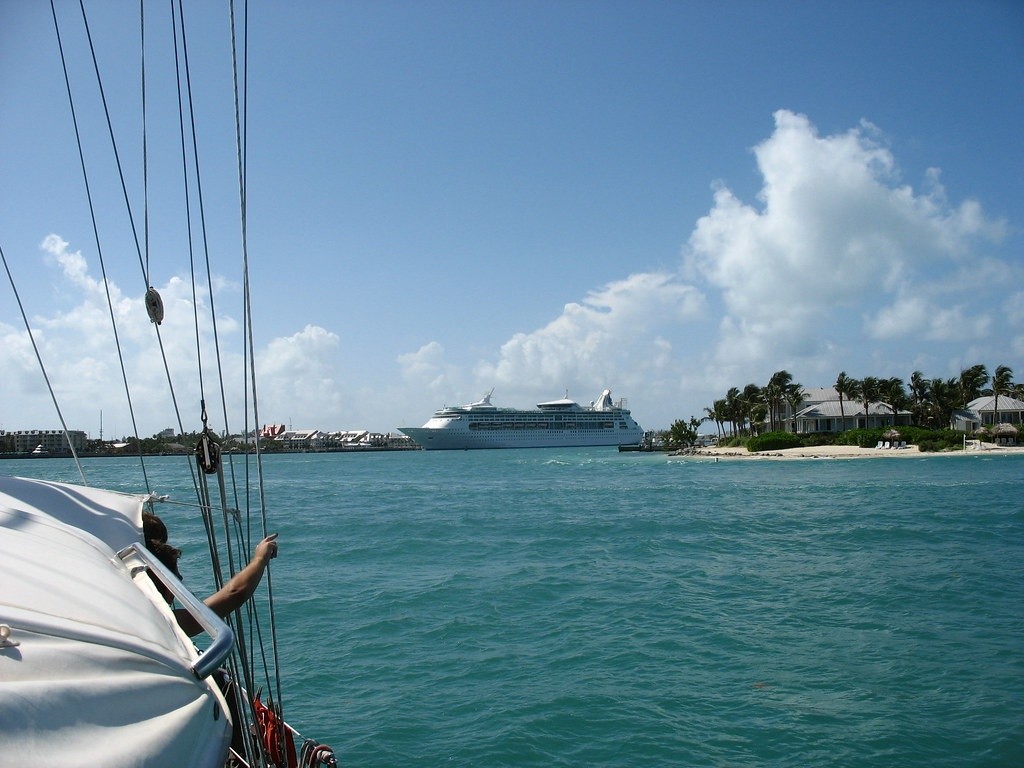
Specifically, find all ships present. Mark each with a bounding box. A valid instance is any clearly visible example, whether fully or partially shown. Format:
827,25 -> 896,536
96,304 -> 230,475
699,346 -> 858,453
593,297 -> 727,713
396,386 -> 645,449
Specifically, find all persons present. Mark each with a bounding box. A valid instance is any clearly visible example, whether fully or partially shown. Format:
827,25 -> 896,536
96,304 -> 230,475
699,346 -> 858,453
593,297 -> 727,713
142,511 -> 278,638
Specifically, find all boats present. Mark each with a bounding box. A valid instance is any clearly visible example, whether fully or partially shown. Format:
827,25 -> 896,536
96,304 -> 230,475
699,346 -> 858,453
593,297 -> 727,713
657,432 -> 712,446
